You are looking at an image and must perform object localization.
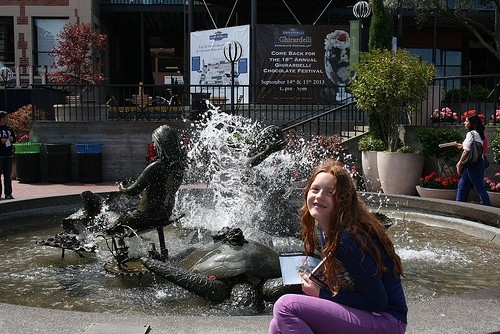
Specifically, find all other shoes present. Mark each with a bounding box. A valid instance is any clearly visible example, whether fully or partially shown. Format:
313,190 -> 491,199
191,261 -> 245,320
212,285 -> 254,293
5,195 -> 15,199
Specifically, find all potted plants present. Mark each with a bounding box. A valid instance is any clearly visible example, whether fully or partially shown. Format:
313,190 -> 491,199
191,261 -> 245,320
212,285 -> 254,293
345,47 -> 436,196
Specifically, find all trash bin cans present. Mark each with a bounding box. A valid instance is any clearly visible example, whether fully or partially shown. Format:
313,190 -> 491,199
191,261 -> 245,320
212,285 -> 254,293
44,143 -> 73,184
75,142 -> 104,182
13,143 -> 41,185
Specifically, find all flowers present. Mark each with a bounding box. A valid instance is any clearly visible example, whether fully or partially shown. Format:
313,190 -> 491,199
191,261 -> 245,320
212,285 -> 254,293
484,172 -> 500,192
461,109 -> 485,123
431,107 -> 459,123
418,173 -> 462,189
491,109 -> 500,121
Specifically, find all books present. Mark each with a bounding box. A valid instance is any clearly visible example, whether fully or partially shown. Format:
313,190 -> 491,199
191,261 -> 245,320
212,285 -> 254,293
307,252 -> 354,297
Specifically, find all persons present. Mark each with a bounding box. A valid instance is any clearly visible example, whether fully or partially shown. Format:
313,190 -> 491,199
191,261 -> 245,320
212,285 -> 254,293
457,113 -> 492,207
482,133 -> 489,168
247,124 -> 288,169
268,162 -> 409,334
0,110 -> 17,200
102,124 -> 193,233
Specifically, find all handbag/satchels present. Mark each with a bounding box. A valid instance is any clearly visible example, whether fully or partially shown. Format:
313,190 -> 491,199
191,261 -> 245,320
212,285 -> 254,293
461,131 -> 483,167
483,155 -> 490,168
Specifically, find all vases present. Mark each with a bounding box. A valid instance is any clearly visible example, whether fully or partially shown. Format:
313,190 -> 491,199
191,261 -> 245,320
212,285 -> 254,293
486,190 -> 500,207
415,185 -> 458,200
